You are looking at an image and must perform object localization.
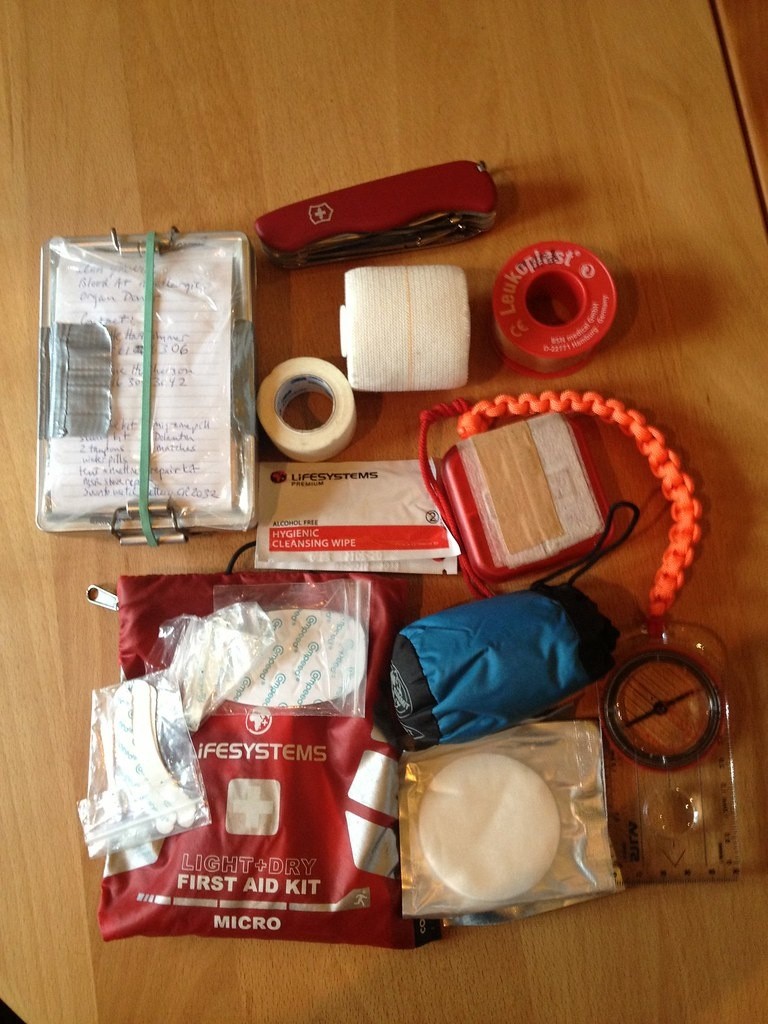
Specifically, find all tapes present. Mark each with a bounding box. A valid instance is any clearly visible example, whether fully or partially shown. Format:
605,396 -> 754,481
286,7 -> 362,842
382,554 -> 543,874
257,356 -> 359,464
489,239 -> 620,381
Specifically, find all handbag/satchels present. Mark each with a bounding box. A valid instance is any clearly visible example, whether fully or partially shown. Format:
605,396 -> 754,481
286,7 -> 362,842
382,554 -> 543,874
82,569 -> 419,951
